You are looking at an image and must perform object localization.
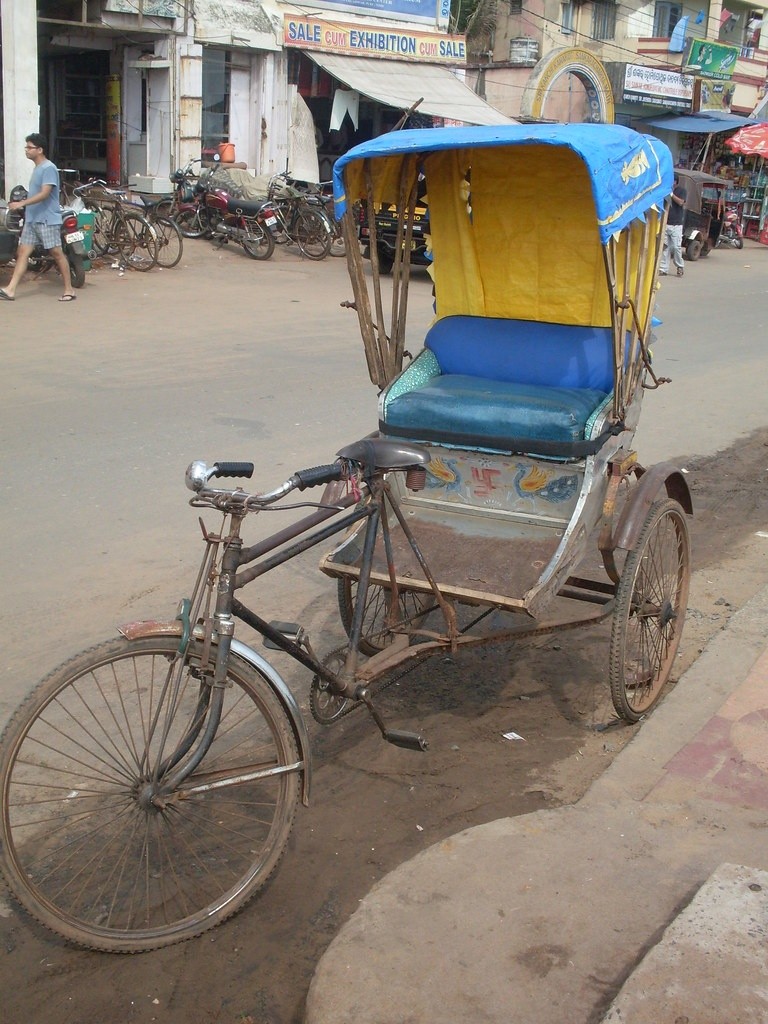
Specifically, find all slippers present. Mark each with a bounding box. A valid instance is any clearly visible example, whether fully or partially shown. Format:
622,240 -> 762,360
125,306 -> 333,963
0,288 -> 15,300
57,292 -> 76,302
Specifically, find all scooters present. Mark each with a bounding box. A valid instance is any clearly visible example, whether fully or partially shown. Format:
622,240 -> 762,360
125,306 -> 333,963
723,193 -> 747,249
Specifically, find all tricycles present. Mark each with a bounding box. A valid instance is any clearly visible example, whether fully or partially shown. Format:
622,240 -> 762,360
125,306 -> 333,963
0,122 -> 695,957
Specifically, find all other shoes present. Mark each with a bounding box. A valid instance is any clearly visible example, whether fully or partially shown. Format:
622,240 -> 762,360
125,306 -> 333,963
658,270 -> 667,276
677,267 -> 683,276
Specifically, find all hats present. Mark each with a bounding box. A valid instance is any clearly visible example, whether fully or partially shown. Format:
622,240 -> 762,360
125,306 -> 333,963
674,173 -> 680,180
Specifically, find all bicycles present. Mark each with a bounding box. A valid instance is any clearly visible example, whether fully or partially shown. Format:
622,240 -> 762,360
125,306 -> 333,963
57,169 -> 184,271
266,158 -> 349,260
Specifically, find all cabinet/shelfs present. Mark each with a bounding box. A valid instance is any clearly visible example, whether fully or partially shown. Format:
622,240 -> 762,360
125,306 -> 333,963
741,185 -> 768,240
55,75 -> 107,182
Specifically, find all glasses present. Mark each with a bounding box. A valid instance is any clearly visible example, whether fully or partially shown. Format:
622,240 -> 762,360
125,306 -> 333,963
24,146 -> 38,151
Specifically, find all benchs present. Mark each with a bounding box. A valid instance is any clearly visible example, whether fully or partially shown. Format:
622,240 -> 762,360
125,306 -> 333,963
377,314 -> 641,527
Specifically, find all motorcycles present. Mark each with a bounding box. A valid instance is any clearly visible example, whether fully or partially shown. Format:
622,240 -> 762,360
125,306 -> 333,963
670,169 -> 728,262
154,152 -> 284,260
1,184 -> 98,288
359,165 -> 472,275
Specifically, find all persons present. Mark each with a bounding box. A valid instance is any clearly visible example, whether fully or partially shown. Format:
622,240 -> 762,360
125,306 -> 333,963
0,132 -> 76,302
660,174 -> 687,276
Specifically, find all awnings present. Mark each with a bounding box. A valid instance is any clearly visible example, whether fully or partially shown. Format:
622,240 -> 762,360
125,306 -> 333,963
634,108 -> 768,158
305,49 -> 528,132
